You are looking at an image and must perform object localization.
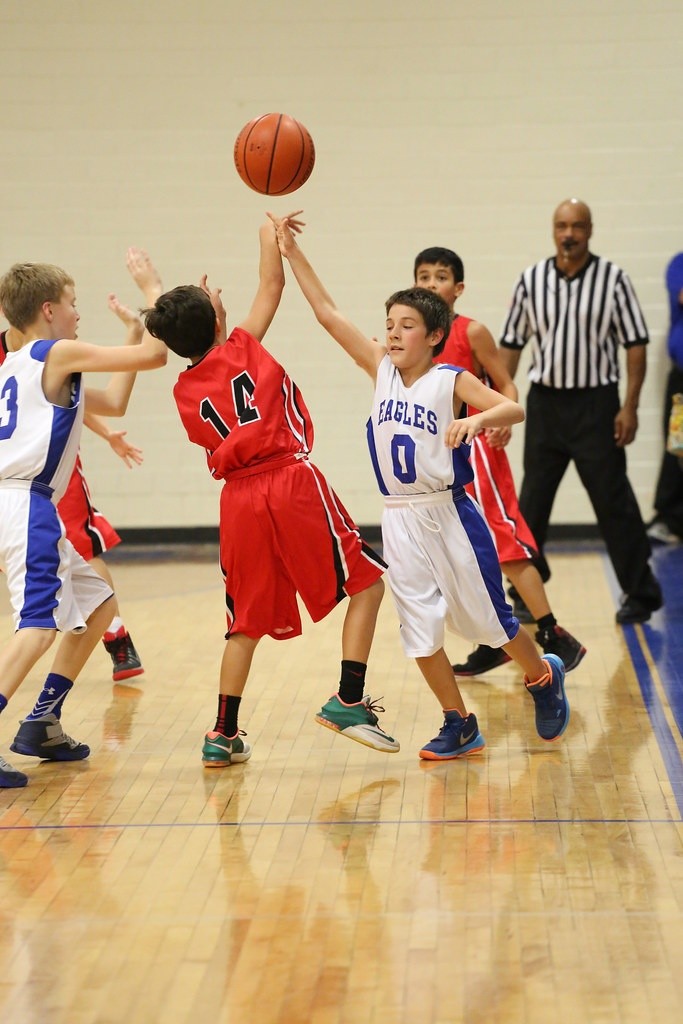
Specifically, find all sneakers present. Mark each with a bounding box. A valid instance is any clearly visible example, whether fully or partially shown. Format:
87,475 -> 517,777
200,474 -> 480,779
535,625 -> 586,672
453,644 -> 515,677
314,693 -> 401,753
10,713 -> 90,760
616,582 -> 664,625
203,730 -> 252,767
0,756 -> 28,787
524,653 -> 570,743
419,709 -> 485,759
103,626 -> 144,682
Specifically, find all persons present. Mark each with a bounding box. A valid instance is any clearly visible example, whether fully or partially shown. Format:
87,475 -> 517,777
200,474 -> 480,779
266,208 -> 569,763
0,319 -> 147,684
372,247 -> 588,682
1,245 -> 169,792
138,210 -> 400,770
495,196 -> 664,626
645,247 -> 683,545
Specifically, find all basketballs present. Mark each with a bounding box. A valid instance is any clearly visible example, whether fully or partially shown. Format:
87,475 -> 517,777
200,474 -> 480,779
234,112 -> 315,195
